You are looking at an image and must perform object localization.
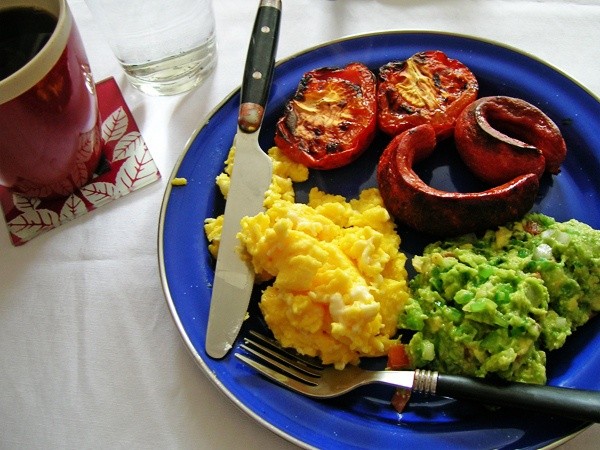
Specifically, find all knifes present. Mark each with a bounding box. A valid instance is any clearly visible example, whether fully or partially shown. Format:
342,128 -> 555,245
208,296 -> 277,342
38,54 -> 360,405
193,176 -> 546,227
204,0 -> 286,359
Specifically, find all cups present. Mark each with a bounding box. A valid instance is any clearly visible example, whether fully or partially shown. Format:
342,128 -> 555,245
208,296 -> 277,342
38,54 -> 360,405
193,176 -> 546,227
1,0 -> 106,203
81,0 -> 218,97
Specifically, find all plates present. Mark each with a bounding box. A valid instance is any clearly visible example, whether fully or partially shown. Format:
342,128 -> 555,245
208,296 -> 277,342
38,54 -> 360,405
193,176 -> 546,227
155,27 -> 600,450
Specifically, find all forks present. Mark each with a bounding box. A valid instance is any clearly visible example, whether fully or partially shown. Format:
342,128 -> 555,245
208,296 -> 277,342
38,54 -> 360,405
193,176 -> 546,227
233,327 -> 599,424
